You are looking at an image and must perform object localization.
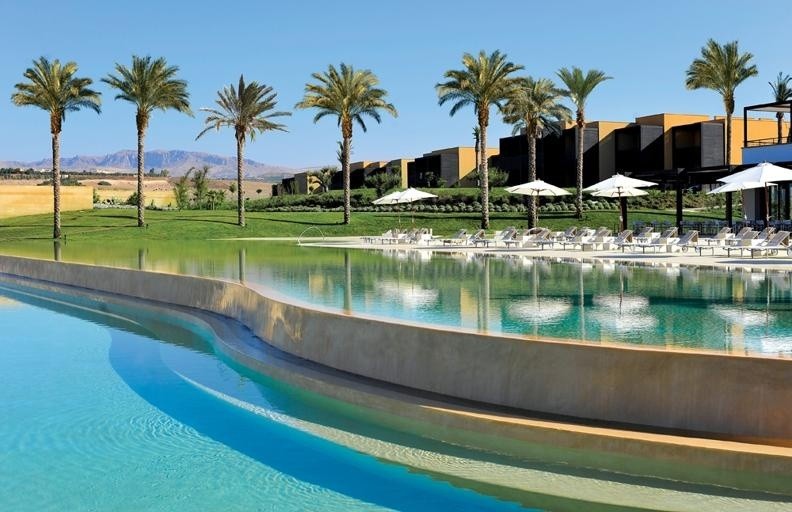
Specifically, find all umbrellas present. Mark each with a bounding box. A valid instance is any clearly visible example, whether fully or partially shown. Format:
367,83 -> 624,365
397,256 -> 440,312
371,190 -> 406,234
593,265 -> 649,318
504,179 -> 573,237
703,281 -> 776,331
393,186 -> 440,231
588,263 -> 660,338
589,187 -> 650,233
717,160 -> 792,238
373,260 -> 421,305
508,267 -> 572,329
705,182 -> 779,228
582,173 -> 659,233
